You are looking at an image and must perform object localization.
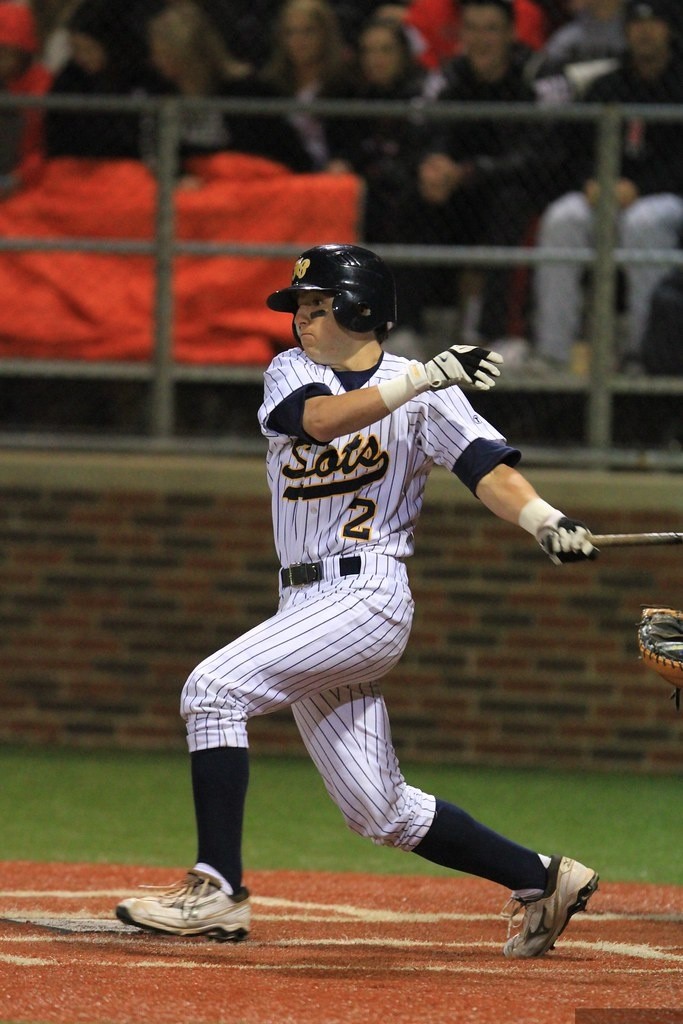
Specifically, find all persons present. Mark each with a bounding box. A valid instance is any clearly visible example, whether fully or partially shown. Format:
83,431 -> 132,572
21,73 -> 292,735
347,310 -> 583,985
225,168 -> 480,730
0,0 -> 683,375
117,245 -> 601,959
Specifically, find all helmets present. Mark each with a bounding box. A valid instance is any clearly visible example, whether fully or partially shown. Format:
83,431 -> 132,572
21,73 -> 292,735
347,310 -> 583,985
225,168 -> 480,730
267,244 -> 397,346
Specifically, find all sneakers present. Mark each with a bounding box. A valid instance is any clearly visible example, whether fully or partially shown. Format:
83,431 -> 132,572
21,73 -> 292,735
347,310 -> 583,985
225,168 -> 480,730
116,869 -> 251,942
501,855 -> 599,957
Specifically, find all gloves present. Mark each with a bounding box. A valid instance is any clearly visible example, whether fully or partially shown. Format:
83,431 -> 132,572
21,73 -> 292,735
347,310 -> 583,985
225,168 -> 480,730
536,510 -> 601,565
408,345 -> 504,393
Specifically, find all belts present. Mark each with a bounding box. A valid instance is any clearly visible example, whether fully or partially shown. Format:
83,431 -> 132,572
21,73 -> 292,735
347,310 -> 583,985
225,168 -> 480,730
281,556 -> 361,588
